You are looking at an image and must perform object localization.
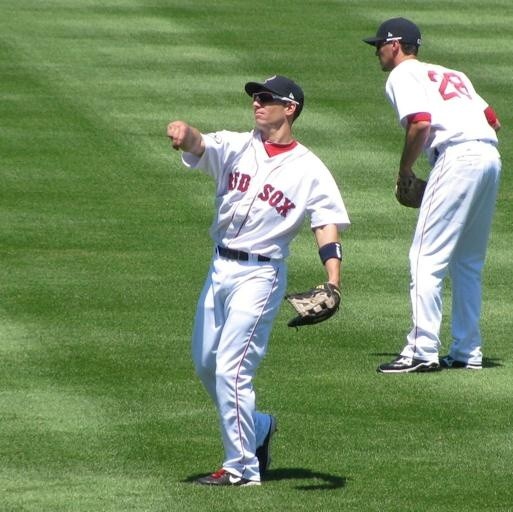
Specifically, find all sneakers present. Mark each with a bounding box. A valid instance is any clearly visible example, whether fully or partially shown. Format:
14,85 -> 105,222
439,355 -> 482,370
377,355 -> 438,374
196,470 -> 261,488
255,413 -> 275,476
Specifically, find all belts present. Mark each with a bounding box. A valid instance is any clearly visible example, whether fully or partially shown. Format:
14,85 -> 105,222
215,246 -> 270,261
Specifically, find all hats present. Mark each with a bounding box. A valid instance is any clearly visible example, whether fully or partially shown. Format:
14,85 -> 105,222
245,74 -> 303,110
361,17 -> 420,46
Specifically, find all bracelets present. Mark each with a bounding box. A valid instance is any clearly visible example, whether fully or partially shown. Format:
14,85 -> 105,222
316,240 -> 346,263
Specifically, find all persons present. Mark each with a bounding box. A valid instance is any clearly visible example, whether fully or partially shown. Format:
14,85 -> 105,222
361,17 -> 503,373
159,75 -> 351,482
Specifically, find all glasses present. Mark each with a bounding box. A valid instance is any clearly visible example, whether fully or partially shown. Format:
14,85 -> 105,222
252,91 -> 299,105
374,36 -> 402,49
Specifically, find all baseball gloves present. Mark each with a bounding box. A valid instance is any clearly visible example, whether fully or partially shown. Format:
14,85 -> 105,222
285,282 -> 340,326
394,173 -> 427,208
